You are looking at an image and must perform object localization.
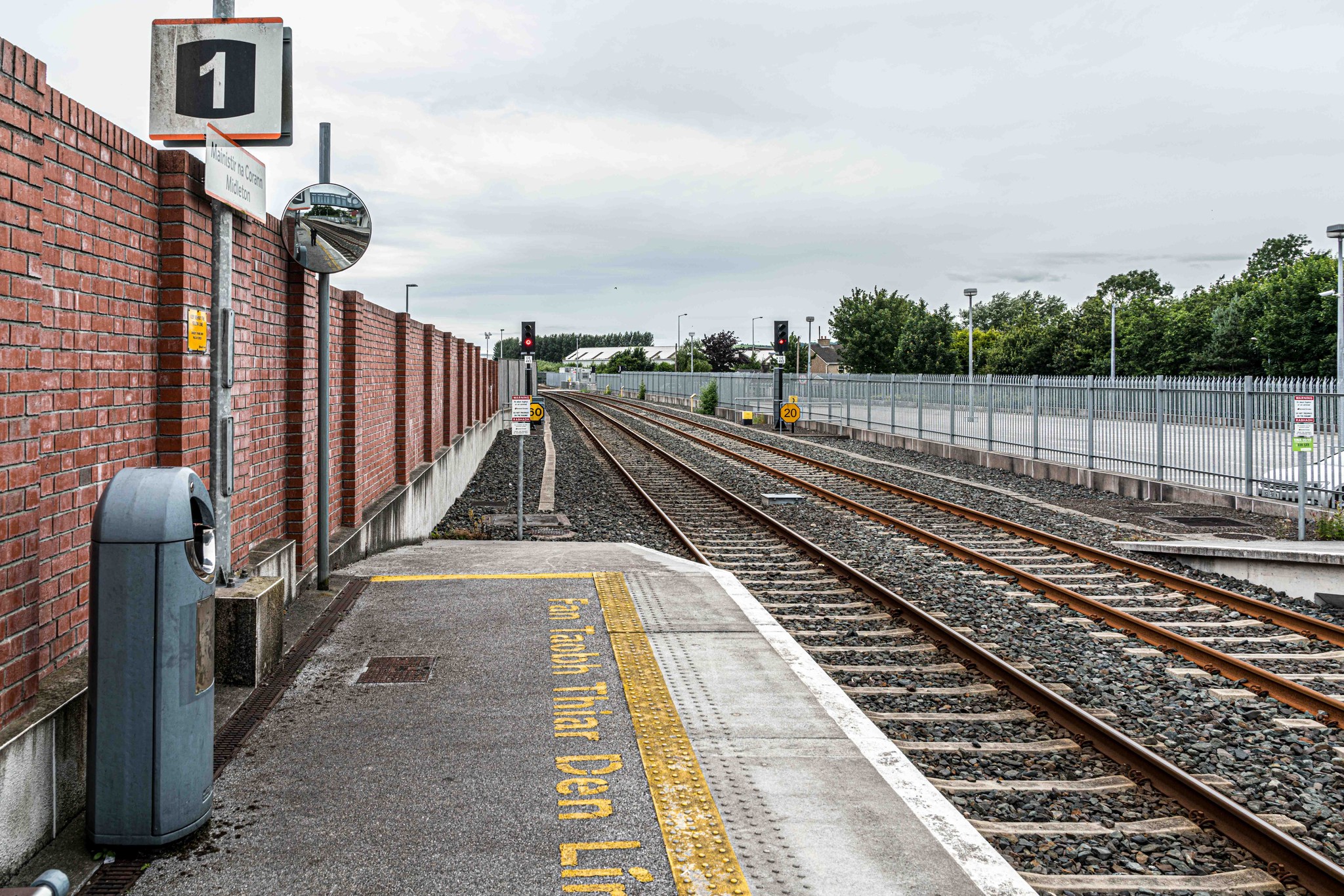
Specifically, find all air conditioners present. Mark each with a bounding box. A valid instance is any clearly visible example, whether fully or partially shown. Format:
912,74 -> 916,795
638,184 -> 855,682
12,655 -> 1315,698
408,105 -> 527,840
565,367 -> 571,371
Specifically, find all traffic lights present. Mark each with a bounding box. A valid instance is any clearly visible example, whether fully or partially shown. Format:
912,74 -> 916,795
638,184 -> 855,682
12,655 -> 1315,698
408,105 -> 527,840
590,366 -> 596,373
521,322 -> 536,352
618,365 -> 625,372
774,321 -> 788,351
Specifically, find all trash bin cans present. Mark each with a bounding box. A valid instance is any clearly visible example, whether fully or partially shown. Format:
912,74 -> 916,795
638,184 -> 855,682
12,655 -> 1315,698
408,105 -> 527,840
83,465 -> 215,856
298,246 -> 308,269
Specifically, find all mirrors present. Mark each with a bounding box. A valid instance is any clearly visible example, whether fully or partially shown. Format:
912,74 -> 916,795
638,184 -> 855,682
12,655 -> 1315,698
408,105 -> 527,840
279,183 -> 372,274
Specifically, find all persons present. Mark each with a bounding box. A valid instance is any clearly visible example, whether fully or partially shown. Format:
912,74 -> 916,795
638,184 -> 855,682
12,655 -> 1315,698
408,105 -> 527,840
310,226 -> 318,246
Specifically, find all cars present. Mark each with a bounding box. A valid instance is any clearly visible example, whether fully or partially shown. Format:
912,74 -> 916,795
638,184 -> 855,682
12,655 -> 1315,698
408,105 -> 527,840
345,218 -> 350,221
335,220 -> 341,224
1258,450 -> 1344,512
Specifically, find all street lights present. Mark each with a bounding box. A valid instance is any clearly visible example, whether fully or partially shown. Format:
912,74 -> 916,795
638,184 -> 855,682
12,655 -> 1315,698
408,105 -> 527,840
1326,224 -> 1344,452
753,317 -> 763,351
406,284 -> 419,313
576,336 -> 582,381
314,208 -> 330,216
501,329 -> 504,358
679,314 -> 695,372
340,208 -> 342,220
806,316 -> 814,373
965,288 -> 979,421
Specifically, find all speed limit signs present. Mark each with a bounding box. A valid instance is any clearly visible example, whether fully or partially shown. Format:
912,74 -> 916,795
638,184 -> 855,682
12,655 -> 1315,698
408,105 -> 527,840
530,403 -> 544,421
799,375 -> 807,384
781,403 -> 800,423
